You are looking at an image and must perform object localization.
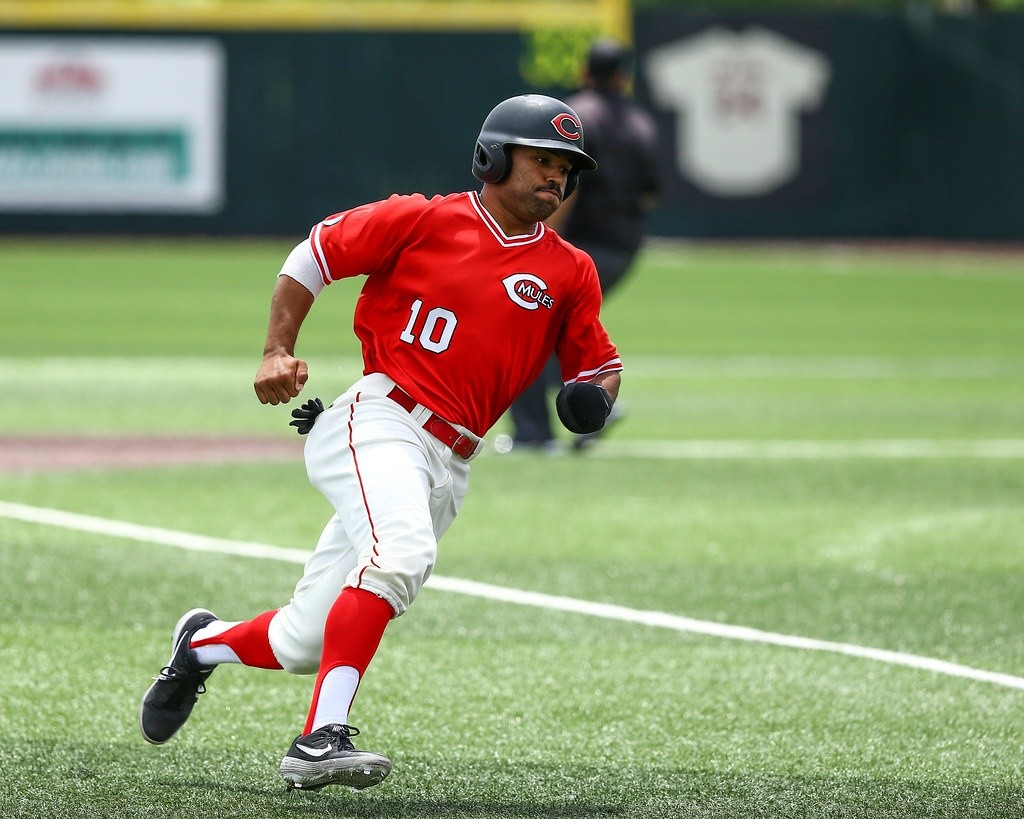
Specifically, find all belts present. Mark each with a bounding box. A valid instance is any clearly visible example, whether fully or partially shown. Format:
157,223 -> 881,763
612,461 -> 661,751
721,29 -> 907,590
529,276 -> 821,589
386,385 -> 478,459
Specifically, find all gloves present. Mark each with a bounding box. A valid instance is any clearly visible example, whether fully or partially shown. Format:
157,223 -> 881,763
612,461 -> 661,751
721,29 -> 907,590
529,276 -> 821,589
288,398 -> 325,435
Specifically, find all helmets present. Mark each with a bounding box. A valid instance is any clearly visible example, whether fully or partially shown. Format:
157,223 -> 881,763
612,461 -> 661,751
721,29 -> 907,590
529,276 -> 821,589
471,94 -> 598,203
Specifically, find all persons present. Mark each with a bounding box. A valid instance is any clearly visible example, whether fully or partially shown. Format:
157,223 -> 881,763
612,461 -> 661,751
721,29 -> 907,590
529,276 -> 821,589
509,42 -> 675,457
142,93 -> 625,792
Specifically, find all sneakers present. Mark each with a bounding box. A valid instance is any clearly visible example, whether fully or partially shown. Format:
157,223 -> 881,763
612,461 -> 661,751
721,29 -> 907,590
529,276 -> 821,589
140,608 -> 220,744
279,723 -> 392,792
